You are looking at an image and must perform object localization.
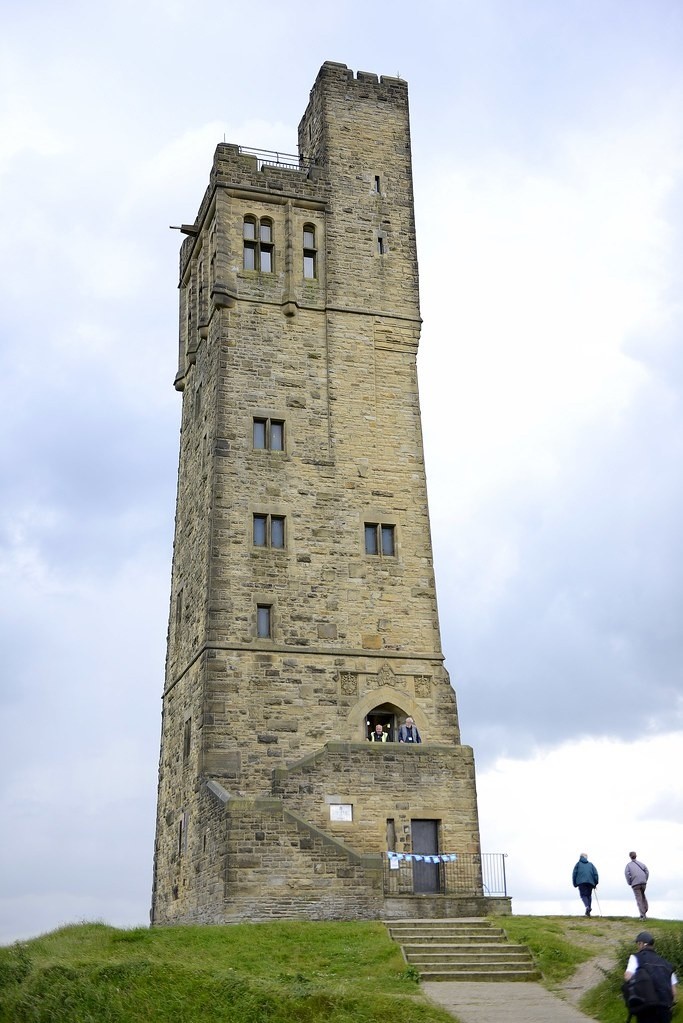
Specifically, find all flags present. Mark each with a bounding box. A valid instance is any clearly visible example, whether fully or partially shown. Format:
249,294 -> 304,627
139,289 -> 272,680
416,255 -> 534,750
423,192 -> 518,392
441,855 -> 449,862
387,852 -> 394,859
432,856 -> 440,863
449,854 -> 457,862
414,855 -> 423,862
396,853 -> 404,860
405,854 -> 412,862
423,856 -> 432,863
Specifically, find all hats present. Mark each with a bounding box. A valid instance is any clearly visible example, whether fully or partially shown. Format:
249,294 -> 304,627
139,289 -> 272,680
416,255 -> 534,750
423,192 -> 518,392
580,853 -> 588,858
630,852 -> 636,857
633,931 -> 653,943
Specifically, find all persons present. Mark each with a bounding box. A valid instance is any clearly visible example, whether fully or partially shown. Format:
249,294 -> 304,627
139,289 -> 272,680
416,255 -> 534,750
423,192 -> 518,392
624,851 -> 649,922
624,931 -> 678,1023
571,852 -> 599,916
365,723 -> 390,743
398,716 -> 421,744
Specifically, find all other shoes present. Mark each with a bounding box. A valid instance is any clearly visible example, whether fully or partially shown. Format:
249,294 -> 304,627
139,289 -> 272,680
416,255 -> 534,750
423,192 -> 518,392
639,918 -> 646,922
585,907 -> 591,916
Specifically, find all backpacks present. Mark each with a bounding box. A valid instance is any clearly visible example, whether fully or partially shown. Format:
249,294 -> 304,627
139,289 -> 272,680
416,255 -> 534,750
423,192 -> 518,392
620,967 -> 659,1016
